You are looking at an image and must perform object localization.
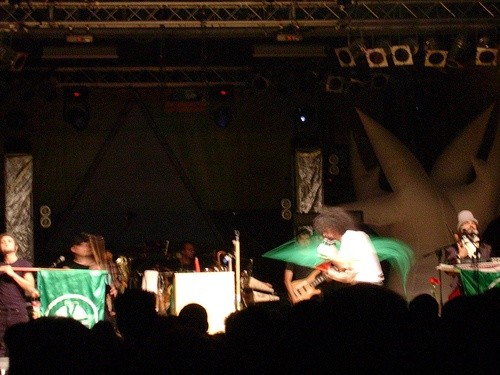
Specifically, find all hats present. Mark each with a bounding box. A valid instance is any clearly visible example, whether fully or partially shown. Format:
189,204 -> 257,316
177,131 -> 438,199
457,210 -> 479,232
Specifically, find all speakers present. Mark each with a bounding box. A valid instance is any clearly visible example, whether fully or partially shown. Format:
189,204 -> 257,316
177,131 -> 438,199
294,104 -> 319,150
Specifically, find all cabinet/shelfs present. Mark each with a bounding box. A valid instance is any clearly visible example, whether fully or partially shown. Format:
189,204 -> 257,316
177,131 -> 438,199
170,271 -> 236,335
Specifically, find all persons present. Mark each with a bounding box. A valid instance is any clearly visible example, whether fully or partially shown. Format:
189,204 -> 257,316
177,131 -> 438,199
312,209 -> 385,287
0,233 -> 38,357
164,242 -> 205,272
59,234 -> 127,323
284,227 -> 329,303
0,278 -> 500,375
441,209 -> 493,299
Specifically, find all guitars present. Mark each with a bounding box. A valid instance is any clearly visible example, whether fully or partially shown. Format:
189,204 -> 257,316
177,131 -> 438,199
287,270 -> 326,304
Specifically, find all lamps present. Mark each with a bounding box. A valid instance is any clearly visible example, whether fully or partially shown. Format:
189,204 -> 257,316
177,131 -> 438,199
0,30 -> 500,139
